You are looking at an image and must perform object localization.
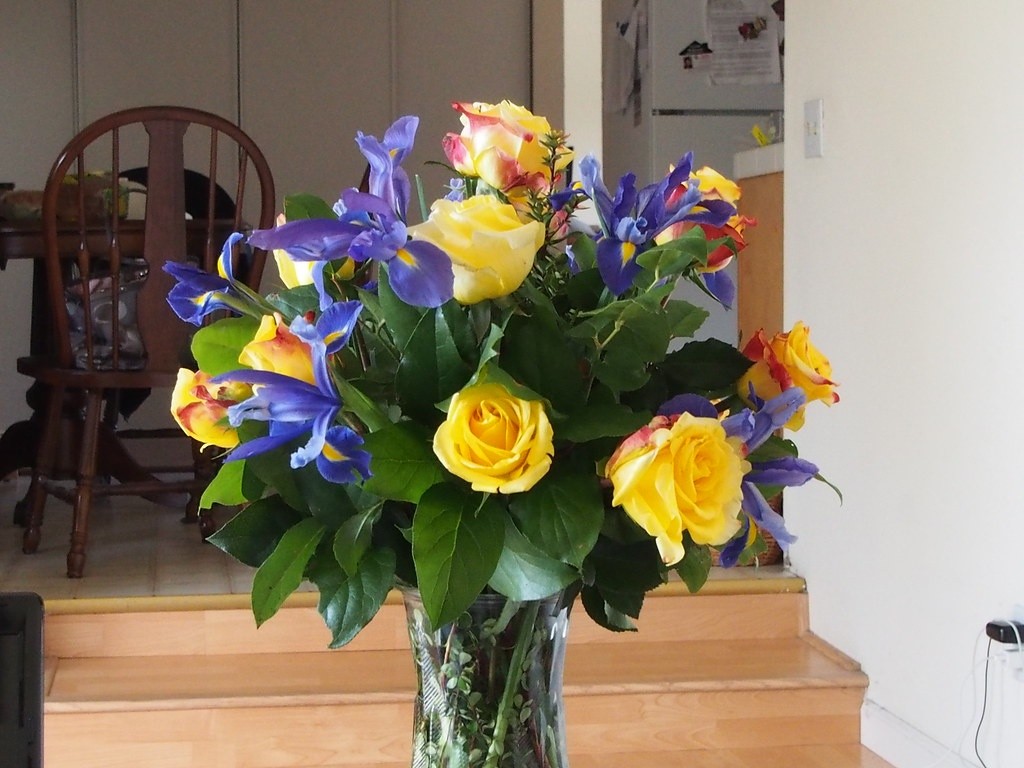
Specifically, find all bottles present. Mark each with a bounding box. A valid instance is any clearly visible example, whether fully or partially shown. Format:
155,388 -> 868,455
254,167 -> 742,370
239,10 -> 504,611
768,111 -> 784,143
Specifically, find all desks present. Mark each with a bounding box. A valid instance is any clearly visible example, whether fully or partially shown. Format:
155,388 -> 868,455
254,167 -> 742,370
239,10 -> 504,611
1,213 -> 250,505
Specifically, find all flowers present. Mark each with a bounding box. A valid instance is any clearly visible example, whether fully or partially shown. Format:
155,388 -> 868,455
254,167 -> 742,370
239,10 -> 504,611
171,98 -> 844,650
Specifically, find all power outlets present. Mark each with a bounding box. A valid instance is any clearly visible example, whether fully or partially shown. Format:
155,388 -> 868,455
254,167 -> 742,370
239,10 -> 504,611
1001,601 -> 1024,683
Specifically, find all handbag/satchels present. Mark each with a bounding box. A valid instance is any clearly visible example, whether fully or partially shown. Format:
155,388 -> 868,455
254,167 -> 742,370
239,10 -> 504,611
62,188 -> 201,371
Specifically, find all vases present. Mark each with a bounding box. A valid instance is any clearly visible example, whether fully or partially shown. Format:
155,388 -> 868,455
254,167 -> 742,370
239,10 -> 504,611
391,577 -> 572,768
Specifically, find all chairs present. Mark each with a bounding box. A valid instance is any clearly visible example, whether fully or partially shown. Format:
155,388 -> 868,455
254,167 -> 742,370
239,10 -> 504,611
95,167 -> 254,504
17,104 -> 276,581
